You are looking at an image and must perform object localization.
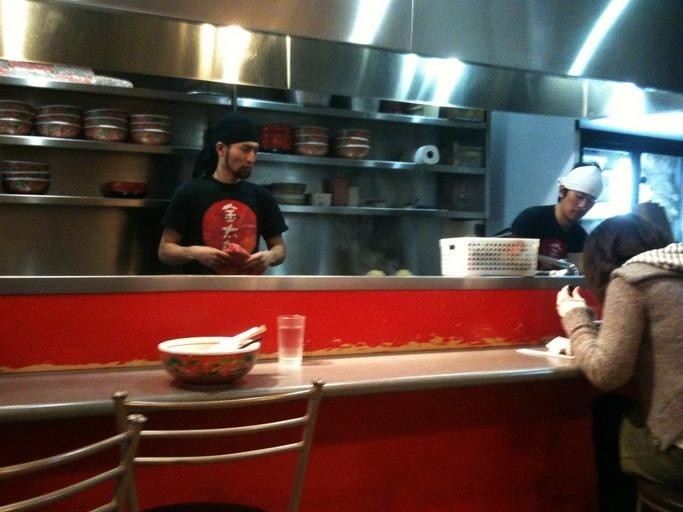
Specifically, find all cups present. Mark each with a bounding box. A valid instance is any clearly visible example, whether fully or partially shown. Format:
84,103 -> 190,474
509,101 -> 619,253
277,314 -> 306,381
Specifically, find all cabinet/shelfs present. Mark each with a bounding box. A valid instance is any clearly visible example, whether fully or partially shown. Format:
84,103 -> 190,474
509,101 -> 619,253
237,96 -> 490,273
1,75 -> 236,276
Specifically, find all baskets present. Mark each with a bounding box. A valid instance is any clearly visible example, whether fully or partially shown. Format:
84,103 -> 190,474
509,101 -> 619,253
438,236 -> 540,278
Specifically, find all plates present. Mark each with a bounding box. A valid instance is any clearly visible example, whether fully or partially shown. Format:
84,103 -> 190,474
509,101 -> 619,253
366,200 -> 387,207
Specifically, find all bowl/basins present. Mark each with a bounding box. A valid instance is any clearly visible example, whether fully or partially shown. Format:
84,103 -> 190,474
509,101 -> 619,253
158,335 -> 263,385
260,122 -> 371,159
1,98 -> 175,145
270,182 -> 308,206
99,181 -> 149,199
0,159 -> 51,194
331,177 -> 360,206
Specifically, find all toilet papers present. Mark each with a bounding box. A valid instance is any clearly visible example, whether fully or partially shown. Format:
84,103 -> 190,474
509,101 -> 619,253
399,144 -> 441,166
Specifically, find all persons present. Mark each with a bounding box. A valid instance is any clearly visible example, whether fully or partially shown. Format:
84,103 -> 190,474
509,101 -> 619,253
157,117 -> 289,273
512,165 -> 603,270
556,217 -> 682,512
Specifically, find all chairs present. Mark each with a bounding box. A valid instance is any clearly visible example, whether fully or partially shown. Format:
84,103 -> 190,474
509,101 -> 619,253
0,412 -> 148,512
112,378 -> 326,511
